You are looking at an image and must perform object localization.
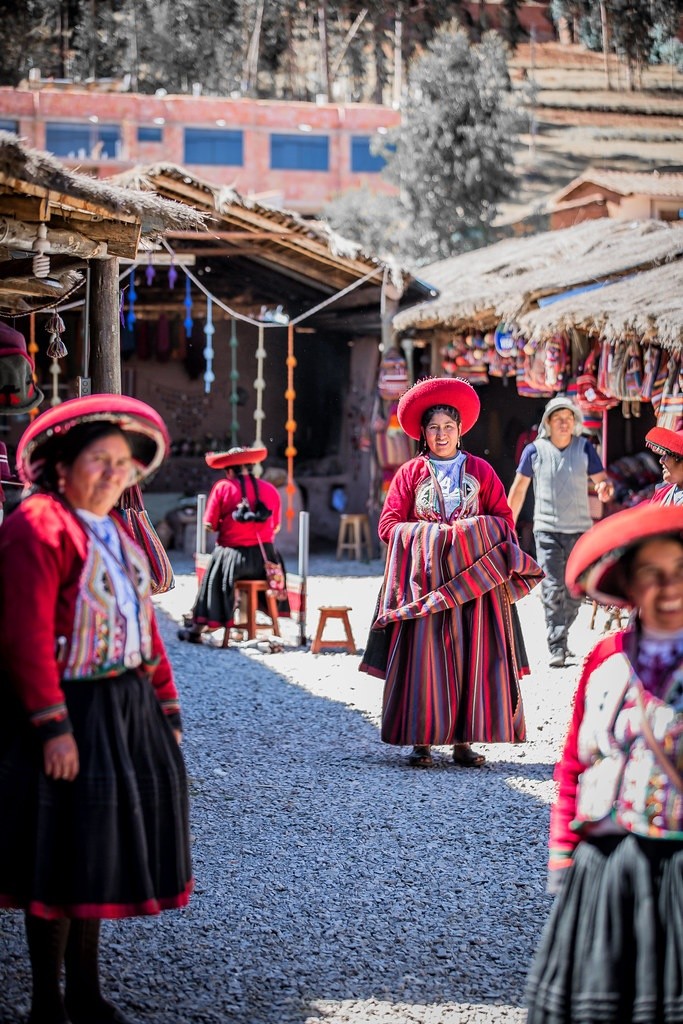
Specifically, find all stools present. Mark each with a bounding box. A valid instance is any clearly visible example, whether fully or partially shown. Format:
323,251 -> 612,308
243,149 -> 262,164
312,607 -> 357,654
223,580 -> 281,647
336,513 -> 374,562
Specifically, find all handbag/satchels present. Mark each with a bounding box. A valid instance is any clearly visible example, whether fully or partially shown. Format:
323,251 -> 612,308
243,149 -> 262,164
264,559 -> 289,601
651,348 -> 683,438
368,346 -> 417,512
494,320 -> 657,413
116,485 -> 176,595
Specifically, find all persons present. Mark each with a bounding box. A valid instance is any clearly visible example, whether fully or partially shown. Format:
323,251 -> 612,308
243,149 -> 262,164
0,392 -> 194,1024
178,446 -> 291,644
521,500 -> 683,1024
357,378 -> 546,767
644,426 -> 683,510
507,397 -> 616,667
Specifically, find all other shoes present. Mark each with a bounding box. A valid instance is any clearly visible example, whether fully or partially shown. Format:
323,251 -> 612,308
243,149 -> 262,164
27,996 -> 131,1024
178,629 -> 202,643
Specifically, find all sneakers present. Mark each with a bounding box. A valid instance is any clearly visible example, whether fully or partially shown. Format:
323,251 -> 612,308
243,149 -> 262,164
550,648 -> 570,665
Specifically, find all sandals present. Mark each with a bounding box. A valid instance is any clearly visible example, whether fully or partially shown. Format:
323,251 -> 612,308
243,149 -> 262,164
409,746 -> 433,766
452,745 -> 485,766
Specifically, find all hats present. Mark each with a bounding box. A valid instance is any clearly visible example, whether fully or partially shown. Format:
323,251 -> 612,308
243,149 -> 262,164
565,505 -> 683,600
17,394 -> 170,492
645,427 -> 683,457
541,396 -> 584,431
397,376 -> 481,441
206,446 -> 268,468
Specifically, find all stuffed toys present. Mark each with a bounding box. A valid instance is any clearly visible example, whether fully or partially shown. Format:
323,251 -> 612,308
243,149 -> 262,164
441,321 -> 683,419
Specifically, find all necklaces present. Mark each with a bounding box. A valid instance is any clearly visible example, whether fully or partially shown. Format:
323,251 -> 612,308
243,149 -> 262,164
69,510 -> 144,602
